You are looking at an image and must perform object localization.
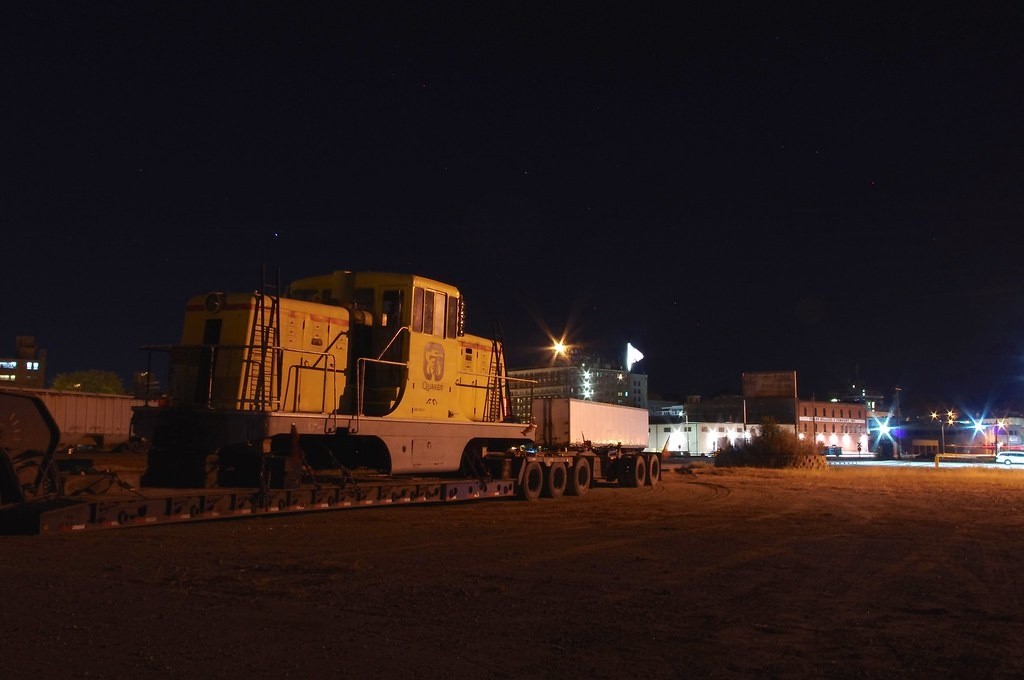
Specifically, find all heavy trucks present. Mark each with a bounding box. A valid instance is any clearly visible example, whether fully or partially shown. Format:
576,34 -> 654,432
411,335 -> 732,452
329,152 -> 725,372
0,270 -> 662,538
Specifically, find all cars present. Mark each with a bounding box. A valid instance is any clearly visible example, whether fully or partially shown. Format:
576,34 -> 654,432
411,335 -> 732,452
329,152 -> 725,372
996,452 -> 1024,465
